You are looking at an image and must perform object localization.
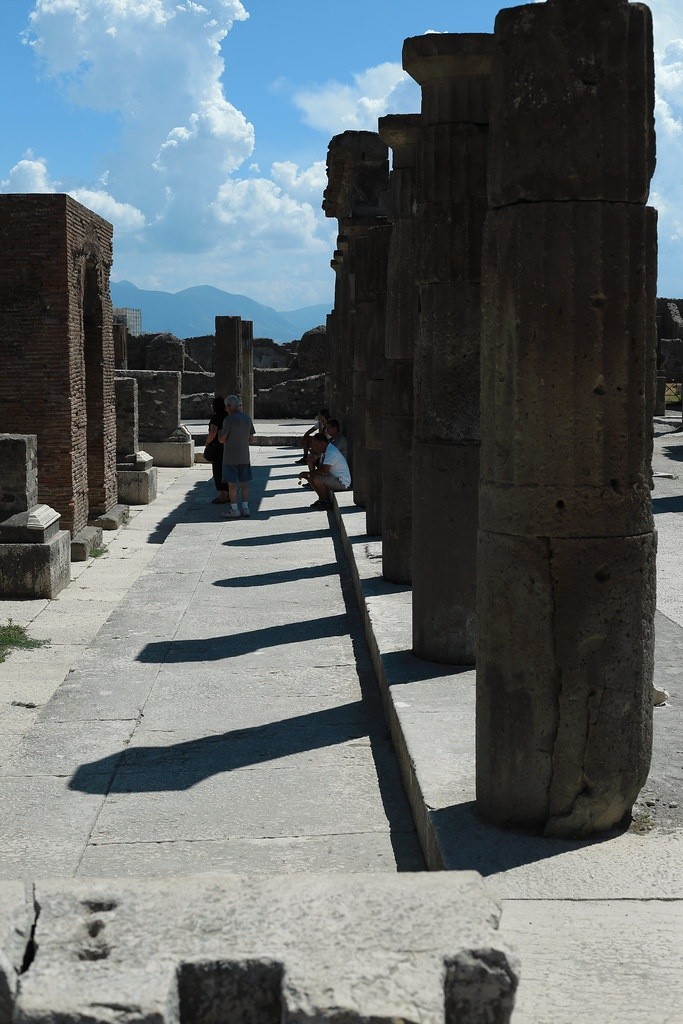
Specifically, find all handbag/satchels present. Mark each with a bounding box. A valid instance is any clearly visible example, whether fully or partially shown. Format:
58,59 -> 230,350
204,443 -> 223,467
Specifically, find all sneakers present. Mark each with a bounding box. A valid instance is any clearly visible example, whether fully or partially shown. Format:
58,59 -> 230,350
296,458 -> 308,464
211,497 -> 228,503
303,482 -> 313,491
310,500 -> 333,510
219,507 -> 250,518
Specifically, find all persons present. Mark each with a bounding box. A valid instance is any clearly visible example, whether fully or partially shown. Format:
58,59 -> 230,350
298,433 -> 351,510
295,409 -> 332,464
205,398 -> 229,504
218,395 -> 256,517
302,419 -> 347,488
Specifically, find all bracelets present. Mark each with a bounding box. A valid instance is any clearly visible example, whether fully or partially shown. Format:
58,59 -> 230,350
205,441 -> 211,444
